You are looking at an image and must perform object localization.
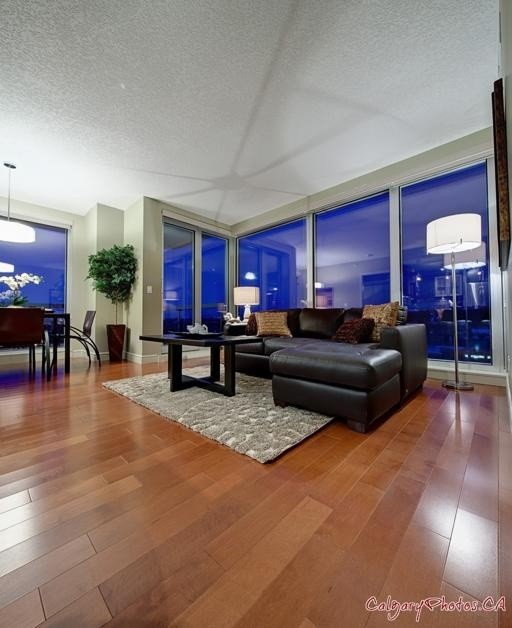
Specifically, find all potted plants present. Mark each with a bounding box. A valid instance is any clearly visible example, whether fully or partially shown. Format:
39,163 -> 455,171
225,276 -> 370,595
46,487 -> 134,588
83,244 -> 137,362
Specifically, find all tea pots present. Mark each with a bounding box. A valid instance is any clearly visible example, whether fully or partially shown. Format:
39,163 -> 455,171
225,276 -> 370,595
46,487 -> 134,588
193,322 -> 208,333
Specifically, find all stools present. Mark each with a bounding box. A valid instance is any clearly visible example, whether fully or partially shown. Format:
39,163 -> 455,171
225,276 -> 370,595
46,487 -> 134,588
269,346 -> 402,433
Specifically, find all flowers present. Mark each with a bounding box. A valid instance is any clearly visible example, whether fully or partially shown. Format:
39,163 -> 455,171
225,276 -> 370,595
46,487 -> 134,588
1,272 -> 42,305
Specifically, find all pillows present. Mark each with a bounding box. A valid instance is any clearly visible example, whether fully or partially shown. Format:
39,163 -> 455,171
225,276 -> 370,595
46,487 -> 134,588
246,301 -> 408,343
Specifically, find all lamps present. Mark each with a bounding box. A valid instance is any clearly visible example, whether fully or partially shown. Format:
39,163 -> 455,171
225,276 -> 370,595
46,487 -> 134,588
0,162 -> 36,243
443,240 -> 487,359
426,212 -> 483,392
233,286 -> 260,323
164,290 -> 183,303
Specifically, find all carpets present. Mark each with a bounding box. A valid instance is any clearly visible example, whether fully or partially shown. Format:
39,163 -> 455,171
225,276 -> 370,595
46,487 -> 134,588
101,362 -> 336,464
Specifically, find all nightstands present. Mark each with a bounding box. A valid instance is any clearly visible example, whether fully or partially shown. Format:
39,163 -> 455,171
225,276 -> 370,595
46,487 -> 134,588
224,322 -> 248,336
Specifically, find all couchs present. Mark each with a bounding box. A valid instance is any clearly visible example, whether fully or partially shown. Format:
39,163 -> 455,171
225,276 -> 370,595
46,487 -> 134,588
223,307 -> 428,398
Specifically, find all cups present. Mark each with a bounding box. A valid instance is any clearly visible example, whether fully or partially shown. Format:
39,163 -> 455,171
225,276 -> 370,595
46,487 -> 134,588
190,328 -> 198,333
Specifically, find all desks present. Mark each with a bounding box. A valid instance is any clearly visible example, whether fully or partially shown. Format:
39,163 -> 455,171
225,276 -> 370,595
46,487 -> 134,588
44,313 -> 70,374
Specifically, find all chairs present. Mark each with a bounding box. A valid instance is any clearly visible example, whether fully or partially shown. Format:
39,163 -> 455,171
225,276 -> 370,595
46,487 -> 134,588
50,311 -> 101,371
0,307 -> 45,380
